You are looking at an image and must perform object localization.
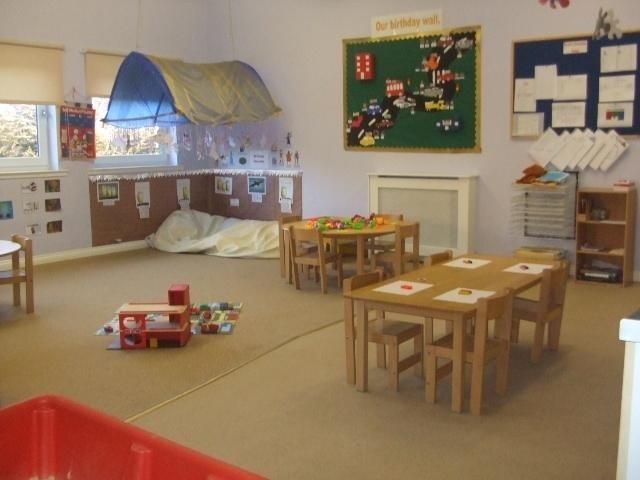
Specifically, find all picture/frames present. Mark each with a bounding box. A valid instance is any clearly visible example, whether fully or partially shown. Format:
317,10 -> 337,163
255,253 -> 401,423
509,27 -> 640,143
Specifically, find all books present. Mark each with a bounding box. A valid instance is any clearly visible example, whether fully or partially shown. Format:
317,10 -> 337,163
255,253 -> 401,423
528,126 -> 629,173
538,171 -> 570,183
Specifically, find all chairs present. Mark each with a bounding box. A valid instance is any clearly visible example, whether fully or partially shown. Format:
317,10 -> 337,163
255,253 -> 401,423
422,286 -> 517,415
426,248 -> 475,345
280,214 -> 319,283
510,245 -> 561,263
340,265 -> 426,393
365,214 -> 404,255
489,262 -> 569,365
287,223 -> 343,295
365,220 -> 422,277
0,234 -> 34,314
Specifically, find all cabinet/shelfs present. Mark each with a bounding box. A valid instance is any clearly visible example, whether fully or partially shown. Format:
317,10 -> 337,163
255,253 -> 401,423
574,186 -> 636,290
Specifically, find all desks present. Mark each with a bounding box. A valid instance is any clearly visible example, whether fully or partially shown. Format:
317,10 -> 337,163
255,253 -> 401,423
281,217 -> 399,285
342,253 -> 556,414
0,239 -> 22,258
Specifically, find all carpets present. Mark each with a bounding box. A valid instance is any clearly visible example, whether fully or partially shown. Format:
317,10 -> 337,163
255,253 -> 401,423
94,301 -> 247,336
